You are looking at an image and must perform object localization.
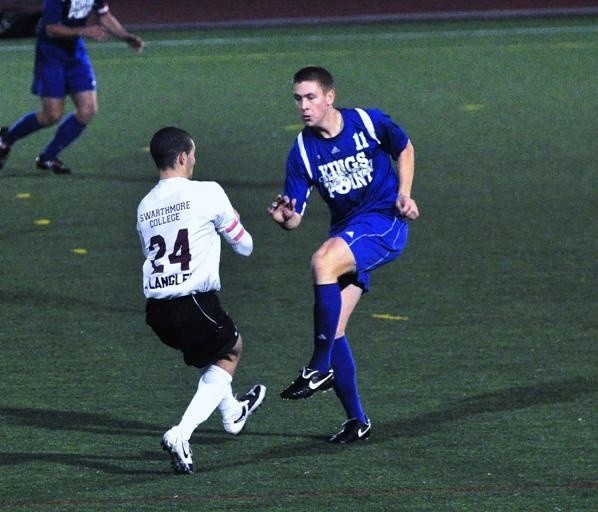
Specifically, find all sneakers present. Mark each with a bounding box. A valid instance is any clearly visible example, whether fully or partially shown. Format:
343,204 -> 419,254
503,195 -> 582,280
0,125 -> 12,171
34,155 -> 73,173
328,414 -> 373,444
221,383 -> 267,435
278,365 -> 336,400
161,425 -> 194,475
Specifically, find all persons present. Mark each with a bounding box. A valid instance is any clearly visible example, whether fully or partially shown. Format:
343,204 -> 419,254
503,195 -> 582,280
134,126 -> 267,475
0,0 -> 148,177
266,65 -> 420,446
